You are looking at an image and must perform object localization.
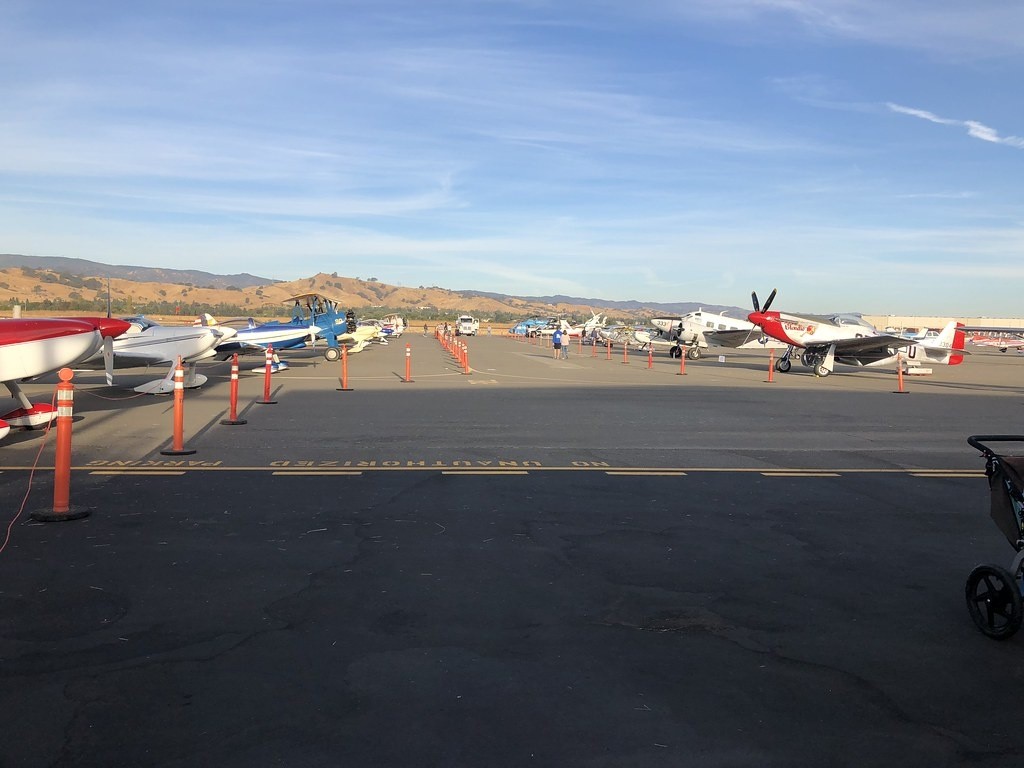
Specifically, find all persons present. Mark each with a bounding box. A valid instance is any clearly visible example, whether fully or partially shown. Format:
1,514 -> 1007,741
590,328 -> 597,345
582,327 -> 586,344
552,325 -> 570,360
487,325 -> 491,336
423,323 -> 428,337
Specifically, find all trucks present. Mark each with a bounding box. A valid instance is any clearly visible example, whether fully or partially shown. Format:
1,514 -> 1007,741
456,315 -> 480,337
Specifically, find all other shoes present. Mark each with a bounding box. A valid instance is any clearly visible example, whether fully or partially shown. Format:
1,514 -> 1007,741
566,354 -> 568,359
553,356 -> 556,359
562,357 -> 564,359
557,357 -> 559,359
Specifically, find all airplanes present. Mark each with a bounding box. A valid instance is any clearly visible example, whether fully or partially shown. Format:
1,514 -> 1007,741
71,315 -> 238,397
0,315 -> 132,441
508,288 -> 1024,378
192,312 -> 321,377
252,292 -> 406,361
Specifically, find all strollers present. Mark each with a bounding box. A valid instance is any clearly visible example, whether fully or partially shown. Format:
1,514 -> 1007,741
967,434 -> 1024,640
